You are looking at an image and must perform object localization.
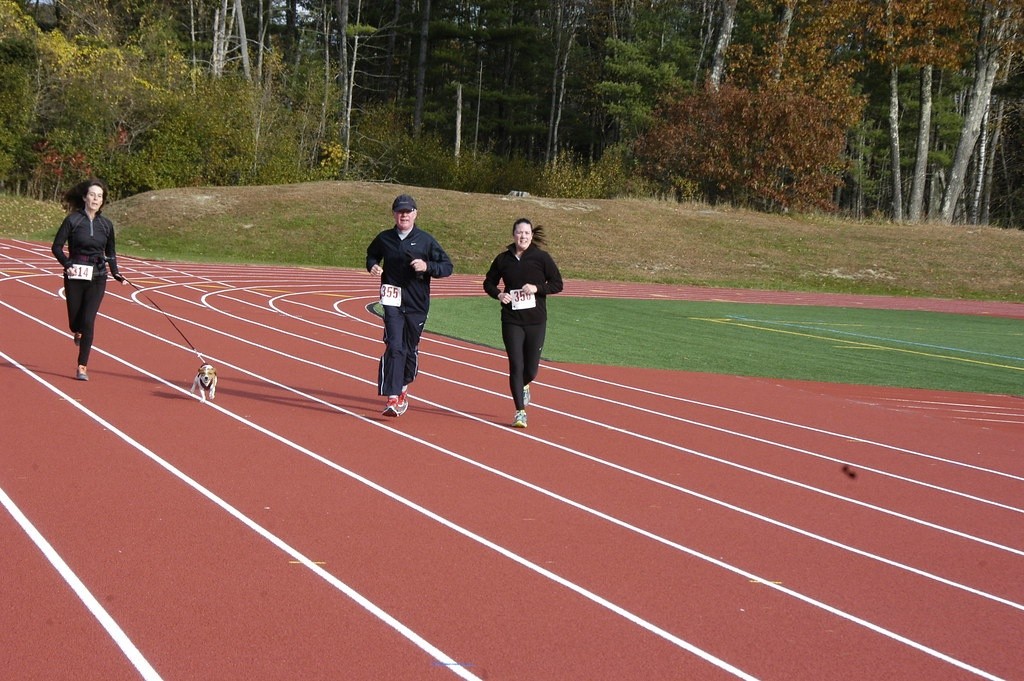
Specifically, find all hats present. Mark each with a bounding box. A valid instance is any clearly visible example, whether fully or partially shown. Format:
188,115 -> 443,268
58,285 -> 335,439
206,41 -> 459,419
393,194 -> 417,211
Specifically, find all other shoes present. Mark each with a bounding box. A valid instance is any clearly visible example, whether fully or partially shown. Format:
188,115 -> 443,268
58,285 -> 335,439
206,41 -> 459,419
76,367 -> 90,380
75,333 -> 81,345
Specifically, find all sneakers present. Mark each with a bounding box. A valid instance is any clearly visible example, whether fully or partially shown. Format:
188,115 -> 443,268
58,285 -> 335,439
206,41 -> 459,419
396,389 -> 408,414
523,385 -> 531,406
512,410 -> 528,427
382,399 -> 401,416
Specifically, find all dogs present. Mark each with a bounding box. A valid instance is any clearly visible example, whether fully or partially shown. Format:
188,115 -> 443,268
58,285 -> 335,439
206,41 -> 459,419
190,362 -> 217,403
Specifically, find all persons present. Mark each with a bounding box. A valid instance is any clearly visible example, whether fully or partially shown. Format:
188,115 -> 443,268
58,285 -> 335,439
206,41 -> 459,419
481,218 -> 563,428
365,194 -> 453,417
51,180 -> 129,381
